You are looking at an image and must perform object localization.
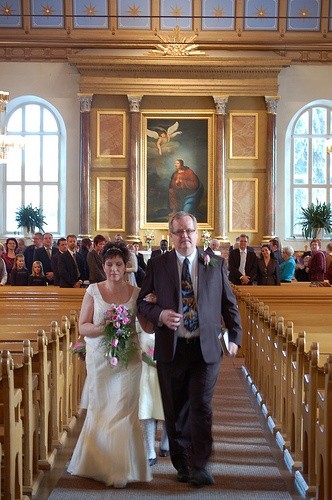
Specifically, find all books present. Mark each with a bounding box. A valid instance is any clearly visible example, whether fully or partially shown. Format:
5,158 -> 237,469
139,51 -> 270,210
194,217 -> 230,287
218,328 -> 229,356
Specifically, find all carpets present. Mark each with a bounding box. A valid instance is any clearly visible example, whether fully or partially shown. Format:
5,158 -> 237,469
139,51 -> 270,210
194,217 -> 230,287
46,358 -> 292,500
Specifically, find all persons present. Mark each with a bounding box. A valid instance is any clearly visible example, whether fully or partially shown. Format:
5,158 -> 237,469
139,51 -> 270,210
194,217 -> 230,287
297,239 -> 326,282
67,240 -> 157,489
150,239 -> 170,259
136,210 -> 244,489
9,254 -> 31,286
52,233 -> 148,288
137,329 -> 169,467
227,234 -> 258,286
28,261 -> 56,286
0,256 -> 7,286
256,244 -> 281,285
203,239 -> 220,256
23,232 -> 43,274
269,239 -> 285,264
278,245 -> 297,283
324,239 -> 332,285
0,237 -> 26,278
34,233 -> 60,285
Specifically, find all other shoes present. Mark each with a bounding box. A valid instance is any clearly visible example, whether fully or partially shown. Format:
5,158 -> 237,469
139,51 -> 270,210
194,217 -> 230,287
176,466 -> 192,482
147,456 -> 158,466
159,448 -> 168,457
190,467 -> 215,484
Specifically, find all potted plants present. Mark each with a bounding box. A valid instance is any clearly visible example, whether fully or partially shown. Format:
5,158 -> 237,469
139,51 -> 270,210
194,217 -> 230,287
15,204 -> 48,239
294,197 -> 332,241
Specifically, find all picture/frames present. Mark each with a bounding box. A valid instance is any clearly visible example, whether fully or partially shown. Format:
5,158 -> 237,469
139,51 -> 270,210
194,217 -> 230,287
139,109 -> 216,231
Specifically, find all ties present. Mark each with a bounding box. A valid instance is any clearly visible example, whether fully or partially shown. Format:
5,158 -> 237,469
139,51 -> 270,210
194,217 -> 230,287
181,258 -> 199,332
73,253 -> 80,277
162,250 -> 164,254
47,248 -> 50,257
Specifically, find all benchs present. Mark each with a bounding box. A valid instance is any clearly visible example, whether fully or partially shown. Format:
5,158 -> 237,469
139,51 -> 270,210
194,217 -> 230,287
0,284 -> 86,500
230,281 -> 332,500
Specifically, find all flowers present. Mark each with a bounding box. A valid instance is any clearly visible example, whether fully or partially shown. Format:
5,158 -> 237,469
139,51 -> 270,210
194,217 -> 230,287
143,229 -> 155,248
99,303 -> 139,371
199,229 -> 212,247
200,252 -> 218,268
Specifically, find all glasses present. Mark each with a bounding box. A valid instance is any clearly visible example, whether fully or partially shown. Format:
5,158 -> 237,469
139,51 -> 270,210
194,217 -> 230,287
172,228 -> 195,237
240,240 -> 247,243
